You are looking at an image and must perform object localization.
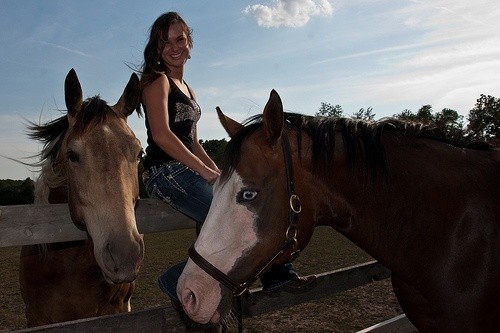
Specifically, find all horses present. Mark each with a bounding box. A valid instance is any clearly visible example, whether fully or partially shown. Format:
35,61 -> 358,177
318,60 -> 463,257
19,67 -> 146,325
176,87 -> 500,333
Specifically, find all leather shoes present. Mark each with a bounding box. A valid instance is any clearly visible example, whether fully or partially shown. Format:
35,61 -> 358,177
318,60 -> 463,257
264,275 -> 317,297
158,276 -> 214,330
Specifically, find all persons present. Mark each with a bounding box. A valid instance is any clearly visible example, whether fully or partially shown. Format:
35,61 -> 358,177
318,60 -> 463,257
136,12 -> 319,333
